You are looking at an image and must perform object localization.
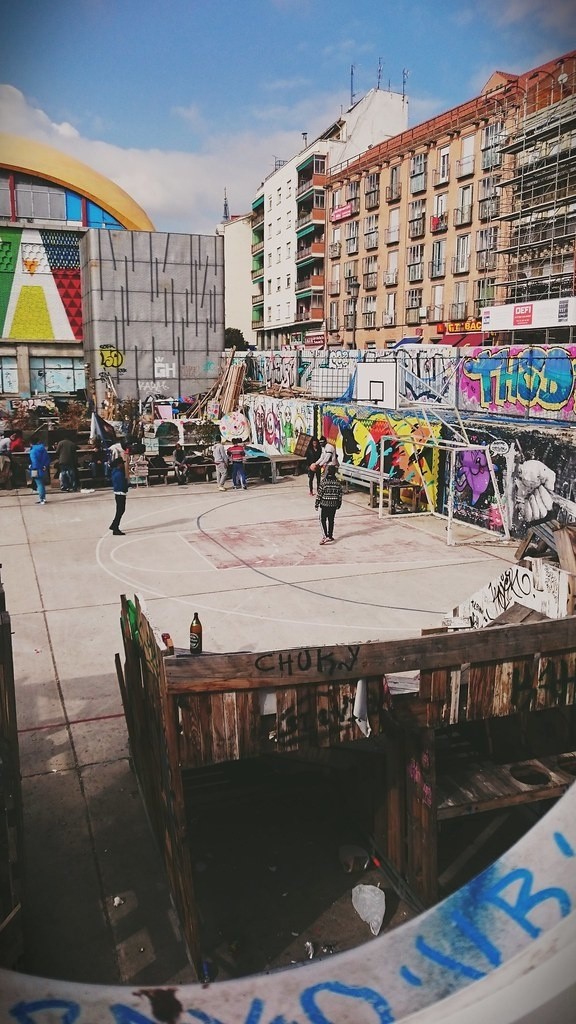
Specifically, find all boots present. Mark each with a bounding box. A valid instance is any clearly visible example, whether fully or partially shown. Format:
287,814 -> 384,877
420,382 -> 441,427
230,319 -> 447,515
109,520 -> 125,535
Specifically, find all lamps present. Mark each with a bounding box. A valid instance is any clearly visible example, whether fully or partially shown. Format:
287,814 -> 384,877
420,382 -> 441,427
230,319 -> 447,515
84,363 -> 90,370
117,368 -> 127,376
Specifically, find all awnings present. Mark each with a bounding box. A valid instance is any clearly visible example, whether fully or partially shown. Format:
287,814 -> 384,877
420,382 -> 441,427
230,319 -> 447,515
437,333 -> 489,347
394,337 -> 420,349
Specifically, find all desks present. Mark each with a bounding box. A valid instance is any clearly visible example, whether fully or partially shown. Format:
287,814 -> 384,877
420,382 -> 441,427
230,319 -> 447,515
369,478 -> 420,515
10,449 -> 97,455
270,455 -> 307,484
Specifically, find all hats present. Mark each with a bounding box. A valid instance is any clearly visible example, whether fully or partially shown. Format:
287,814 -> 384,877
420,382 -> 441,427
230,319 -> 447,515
114,457 -> 126,467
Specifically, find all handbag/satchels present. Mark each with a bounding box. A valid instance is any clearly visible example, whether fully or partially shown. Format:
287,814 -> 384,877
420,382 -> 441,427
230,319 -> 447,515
31,470 -> 38,478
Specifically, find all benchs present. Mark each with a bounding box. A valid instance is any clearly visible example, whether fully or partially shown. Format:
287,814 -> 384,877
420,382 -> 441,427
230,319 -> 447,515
335,462 -> 390,493
78,464 -> 216,486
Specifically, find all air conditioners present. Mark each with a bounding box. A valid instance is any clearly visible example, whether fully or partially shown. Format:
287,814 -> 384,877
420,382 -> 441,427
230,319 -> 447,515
383,315 -> 391,324
386,275 -> 393,283
420,308 -> 426,317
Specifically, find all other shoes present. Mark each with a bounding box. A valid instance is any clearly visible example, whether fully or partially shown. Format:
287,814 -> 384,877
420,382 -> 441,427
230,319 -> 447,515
217,487 -> 226,491
34,499 -> 47,505
320,537 -> 326,545
243,486 -> 247,489
309,491 -> 313,497
233,486 -> 237,489
328,537 -> 334,541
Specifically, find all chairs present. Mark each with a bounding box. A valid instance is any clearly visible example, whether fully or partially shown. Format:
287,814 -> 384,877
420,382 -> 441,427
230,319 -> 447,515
0,453 -> 14,489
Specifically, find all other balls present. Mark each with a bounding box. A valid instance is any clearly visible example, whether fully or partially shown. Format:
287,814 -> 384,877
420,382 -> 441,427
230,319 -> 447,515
310,463 -> 318,472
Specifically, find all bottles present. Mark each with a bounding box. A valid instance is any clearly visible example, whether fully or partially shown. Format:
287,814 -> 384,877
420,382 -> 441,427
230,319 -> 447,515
190,612 -> 202,655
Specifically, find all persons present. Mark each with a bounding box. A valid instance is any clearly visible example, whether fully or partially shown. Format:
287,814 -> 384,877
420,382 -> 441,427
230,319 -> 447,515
314,464 -> 343,545
212,435 -> 228,490
89,445 -> 108,480
109,457 -> 128,535
172,443 -> 188,485
28,437 -> 49,504
306,436 -> 336,495
227,438 -> 247,490
0,430 -> 25,457
55,436 -> 78,491
